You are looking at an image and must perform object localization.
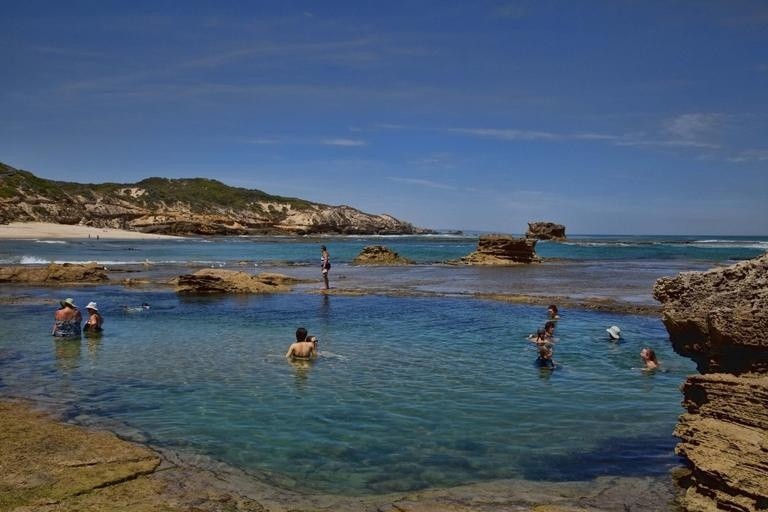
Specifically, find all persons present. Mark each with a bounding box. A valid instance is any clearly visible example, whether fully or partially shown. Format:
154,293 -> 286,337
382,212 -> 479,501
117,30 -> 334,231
306,336 -> 318,350
527,305 -> 560,370
630,348 -> 659,371
320,245 -> 331,289
83,302 -> 101,329
285,327 -> 317,358
119,303 -> 150,314
52,298 -> 82,337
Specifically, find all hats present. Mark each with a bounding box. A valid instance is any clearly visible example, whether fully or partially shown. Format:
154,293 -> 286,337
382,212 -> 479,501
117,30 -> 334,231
85,301 -> 99,311
65,298 -> 79,308
